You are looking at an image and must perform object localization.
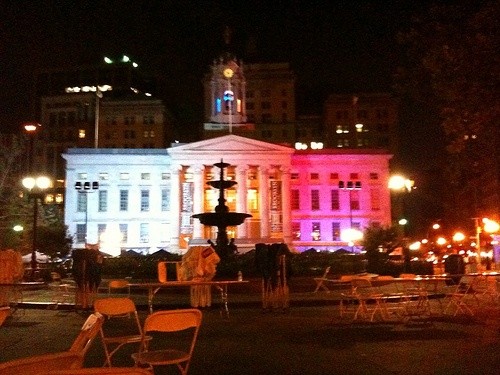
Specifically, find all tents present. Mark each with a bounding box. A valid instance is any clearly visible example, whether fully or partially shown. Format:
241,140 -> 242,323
72,248 -> 103,313
183,246 -> 221,309
255,243 -> 291,311
0,249 -> 23,304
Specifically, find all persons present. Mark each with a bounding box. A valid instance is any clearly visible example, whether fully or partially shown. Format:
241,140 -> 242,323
208,239 -> 214,247
229,238 -> 239,253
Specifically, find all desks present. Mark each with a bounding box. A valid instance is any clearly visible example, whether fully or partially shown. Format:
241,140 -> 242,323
0,281 -> 45,322
466,274 -> 500,306
371,280 -> 412,318
131,281 -> 251,320
415,278 -> 450,316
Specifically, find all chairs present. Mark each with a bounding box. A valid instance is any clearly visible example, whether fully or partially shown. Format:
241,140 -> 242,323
108,281 -> 131,319
94,297 -> 143,366
50,366 -> 155,375
71,311 -> 105,363
50,271 -> 80,310
328,271 -> 500,327
0,351 -> 80,375
131,309 -> 202,375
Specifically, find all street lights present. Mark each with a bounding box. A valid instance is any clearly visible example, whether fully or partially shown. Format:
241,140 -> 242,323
66,84 -> 112,147
122,54 -> 152,147
338,180 -> 362,252
22,175 -> 64,281
74,181 -> 99,248
223,68 -> 233,134
103,57 -> 116,96
25,123 -> 36,203
426,223 -> 440,251
